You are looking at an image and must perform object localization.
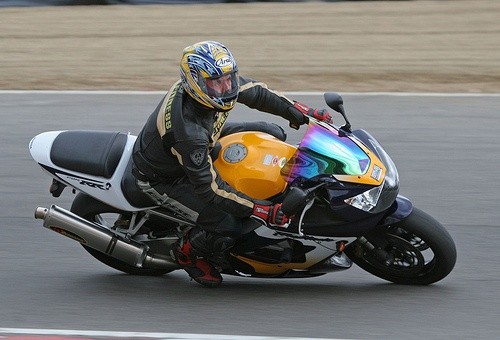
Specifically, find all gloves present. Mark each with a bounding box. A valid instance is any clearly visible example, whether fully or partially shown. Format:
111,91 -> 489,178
288,100 -> 334,129
250,199 -> 295,231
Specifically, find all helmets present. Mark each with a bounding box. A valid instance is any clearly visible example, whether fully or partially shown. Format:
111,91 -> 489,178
178,40 -> 240,112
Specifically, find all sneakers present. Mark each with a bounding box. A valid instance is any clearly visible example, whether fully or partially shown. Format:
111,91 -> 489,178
168,232 -> 224,288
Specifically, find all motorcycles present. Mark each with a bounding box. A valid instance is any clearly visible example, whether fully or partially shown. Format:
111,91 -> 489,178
27,90 -> 457,289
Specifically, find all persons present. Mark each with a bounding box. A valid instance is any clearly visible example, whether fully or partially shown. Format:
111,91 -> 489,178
130,41 -> 335,288
